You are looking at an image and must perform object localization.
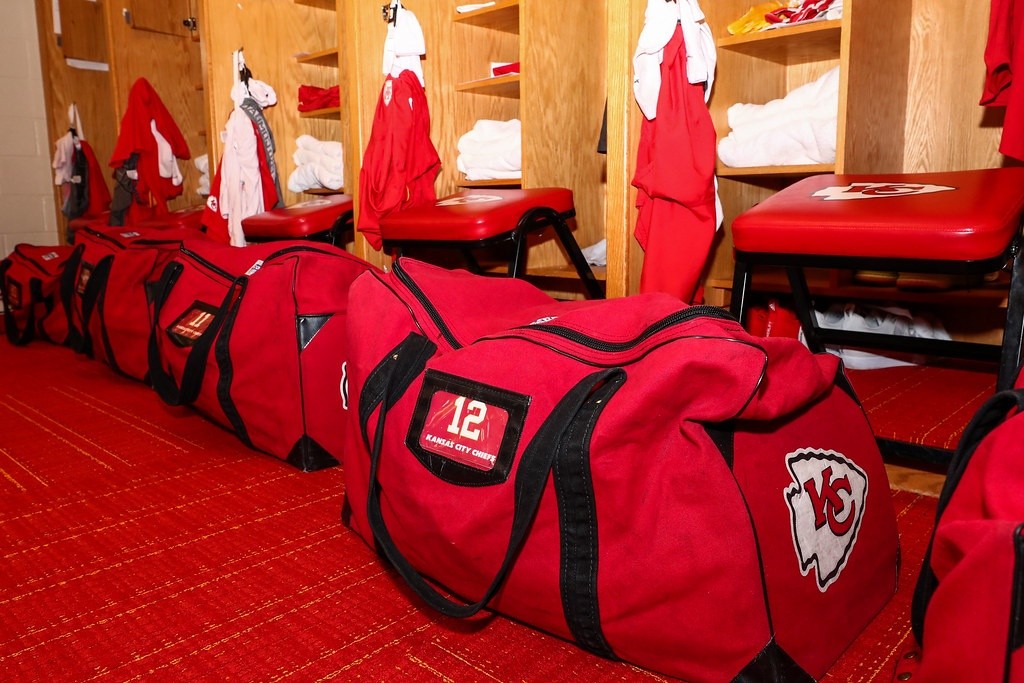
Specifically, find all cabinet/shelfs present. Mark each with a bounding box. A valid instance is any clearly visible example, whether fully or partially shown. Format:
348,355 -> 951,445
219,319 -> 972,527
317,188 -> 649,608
33,0 -> 1023,498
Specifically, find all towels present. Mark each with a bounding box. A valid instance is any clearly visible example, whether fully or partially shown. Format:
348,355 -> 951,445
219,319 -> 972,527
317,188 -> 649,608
285,133 -> 346,190
456,119 -> 522,181
977,0 -> 1024,161
630,0 -> 719,305
106,76 -> 191,216
354,8 -> 440,252
718,65 -> 841,167
201,100 -> 284,250
193,155 -> 211,196
51,132 -> 112,233
296,85 -> 340,112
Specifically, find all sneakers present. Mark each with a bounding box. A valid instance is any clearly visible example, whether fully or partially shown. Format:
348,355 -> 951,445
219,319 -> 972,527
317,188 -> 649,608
798,300 -> 845,358
838,303 -> 953,371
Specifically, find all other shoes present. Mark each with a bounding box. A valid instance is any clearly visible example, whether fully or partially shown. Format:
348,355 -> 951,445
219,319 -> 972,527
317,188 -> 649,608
746,305 -> 765,337
765,298 -> 800,341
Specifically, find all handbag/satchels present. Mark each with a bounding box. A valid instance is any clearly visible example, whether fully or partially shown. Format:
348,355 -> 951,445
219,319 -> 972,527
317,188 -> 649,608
41,224 -> 220,390
148,238 -> 386,474
340,255 -> 903,683
0,243 -> 71,348
890,365 -> 1024,682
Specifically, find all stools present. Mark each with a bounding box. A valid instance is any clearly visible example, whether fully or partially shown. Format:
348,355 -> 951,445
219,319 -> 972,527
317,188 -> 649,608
68,209 -> 113,231
379,185 -> 606,301
727,165 -> 1024,476
242,194 -> 355,246
137,202 -> 207,229
